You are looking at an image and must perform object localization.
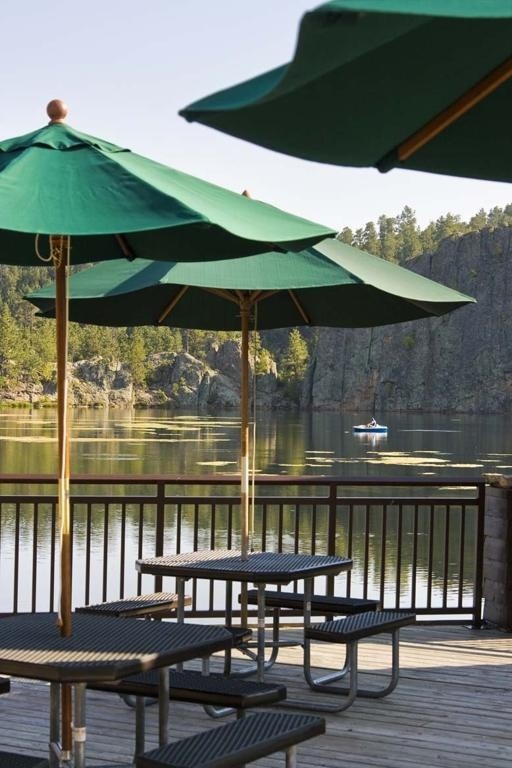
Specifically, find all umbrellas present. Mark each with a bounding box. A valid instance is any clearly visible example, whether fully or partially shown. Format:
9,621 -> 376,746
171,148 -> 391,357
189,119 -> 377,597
19,186 -> 478,654
175,0 -> 512,185
0,99 -> 340,768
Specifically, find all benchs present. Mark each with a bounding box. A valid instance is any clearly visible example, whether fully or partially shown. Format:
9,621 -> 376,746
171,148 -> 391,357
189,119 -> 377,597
225,625 -> 254,646
303,610 -> 417,711
0,748 -> 50,768
237,590 -> 379,670
0,676 -> 11,694
120,668 -> 287,758
74,592 -> 191,624
134,712 -> 326,768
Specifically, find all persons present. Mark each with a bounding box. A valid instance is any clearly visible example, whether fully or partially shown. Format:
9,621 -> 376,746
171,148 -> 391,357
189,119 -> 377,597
365,417 -> 376,428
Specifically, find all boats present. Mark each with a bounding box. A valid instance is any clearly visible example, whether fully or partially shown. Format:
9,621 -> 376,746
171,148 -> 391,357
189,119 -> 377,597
353,428 -> 389,440
351,423 -> 388,433
